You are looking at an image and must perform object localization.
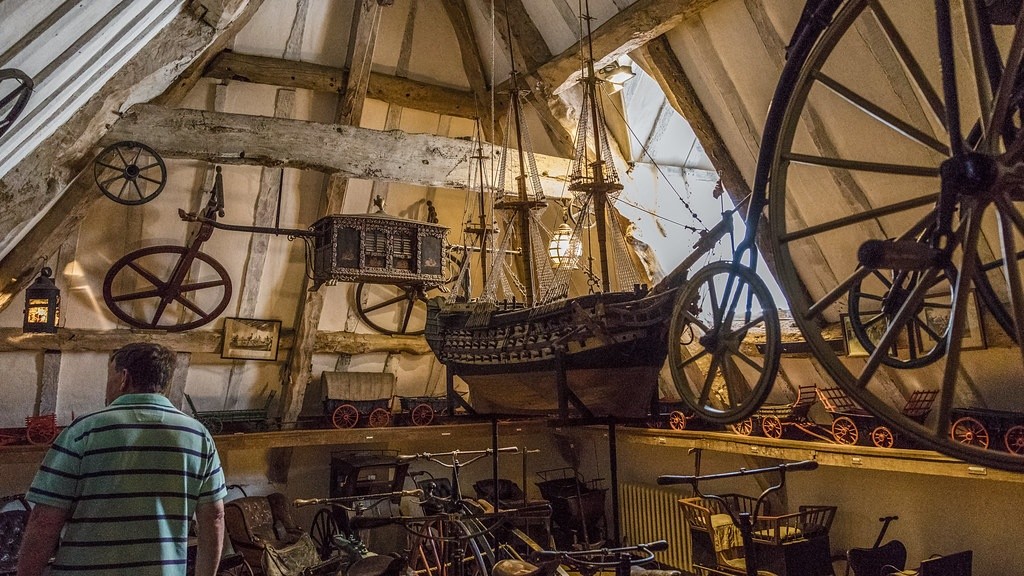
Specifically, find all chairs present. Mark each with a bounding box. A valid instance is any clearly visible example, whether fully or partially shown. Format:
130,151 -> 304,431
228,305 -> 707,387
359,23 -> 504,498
471,479 -> 551,517
223,493 -> 304,568
417,477 -> 474,529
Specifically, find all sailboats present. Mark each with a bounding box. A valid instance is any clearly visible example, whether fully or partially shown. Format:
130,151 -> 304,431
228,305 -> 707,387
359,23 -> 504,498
424,0 -> 702,424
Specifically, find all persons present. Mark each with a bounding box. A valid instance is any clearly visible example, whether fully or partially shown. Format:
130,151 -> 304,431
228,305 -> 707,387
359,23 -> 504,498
17,342 -> 228,576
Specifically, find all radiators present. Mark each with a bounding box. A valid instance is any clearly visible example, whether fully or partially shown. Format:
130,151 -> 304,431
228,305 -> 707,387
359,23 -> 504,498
620,480 -> 723,576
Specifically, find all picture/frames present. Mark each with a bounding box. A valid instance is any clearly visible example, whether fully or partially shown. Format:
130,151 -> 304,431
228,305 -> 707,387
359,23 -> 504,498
915,287 -> 987,354
220,317 -> 282,361
839,310 -> 898,358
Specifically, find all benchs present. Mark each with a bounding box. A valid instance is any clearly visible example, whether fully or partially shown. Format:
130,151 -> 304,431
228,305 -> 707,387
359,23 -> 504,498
749,505 -> 839,576
677,493 -> 770,568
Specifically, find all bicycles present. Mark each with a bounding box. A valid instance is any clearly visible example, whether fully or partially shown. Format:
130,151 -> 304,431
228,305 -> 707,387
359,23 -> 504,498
101,0 -> 1024,576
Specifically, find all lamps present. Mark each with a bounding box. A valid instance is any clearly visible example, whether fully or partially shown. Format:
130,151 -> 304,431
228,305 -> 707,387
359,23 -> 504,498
603,61 -> 636,85
547,200 -> 583,270
24,267 -> 62,333
